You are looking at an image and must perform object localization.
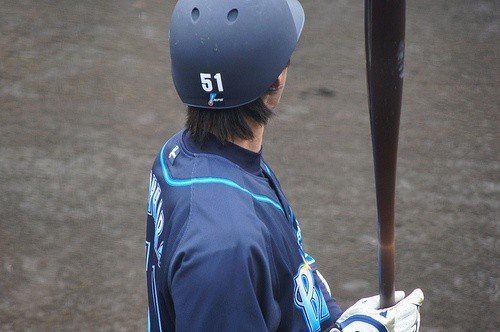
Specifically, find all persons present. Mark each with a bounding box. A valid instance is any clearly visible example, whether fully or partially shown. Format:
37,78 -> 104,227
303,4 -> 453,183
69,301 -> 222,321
144,0 -> 424,332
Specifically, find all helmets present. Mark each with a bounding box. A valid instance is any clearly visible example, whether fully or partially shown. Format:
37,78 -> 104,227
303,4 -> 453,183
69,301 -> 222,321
169,0 -> 305,108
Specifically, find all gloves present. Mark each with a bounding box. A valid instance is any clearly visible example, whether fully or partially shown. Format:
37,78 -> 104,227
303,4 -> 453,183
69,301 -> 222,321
335,287 -> 425,332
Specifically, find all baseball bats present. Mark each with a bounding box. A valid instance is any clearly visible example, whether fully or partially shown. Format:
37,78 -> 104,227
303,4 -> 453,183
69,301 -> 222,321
363,0 -> 406,308
